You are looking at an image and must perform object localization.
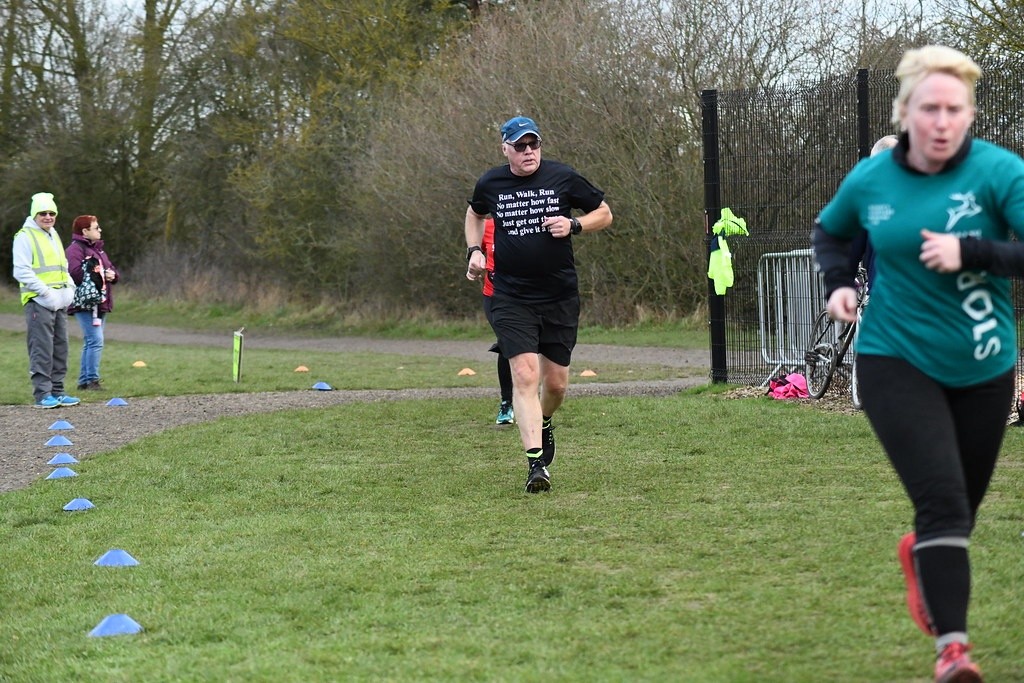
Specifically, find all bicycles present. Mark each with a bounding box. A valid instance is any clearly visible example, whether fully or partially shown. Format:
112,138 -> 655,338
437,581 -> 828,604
804,260 -> 870,409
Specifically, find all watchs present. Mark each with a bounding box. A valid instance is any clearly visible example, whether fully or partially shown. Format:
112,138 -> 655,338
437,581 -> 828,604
466,246 -> 480,260
571,217 -> 582,235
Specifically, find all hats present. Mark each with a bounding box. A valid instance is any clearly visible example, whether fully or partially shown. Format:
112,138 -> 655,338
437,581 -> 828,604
501,117 -> 542,142
30,192 -> 58,220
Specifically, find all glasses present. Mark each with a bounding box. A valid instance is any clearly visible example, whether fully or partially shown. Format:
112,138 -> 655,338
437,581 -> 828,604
81,225 -> 101,230
37,212 -> 56,217
503,141 -> 542,152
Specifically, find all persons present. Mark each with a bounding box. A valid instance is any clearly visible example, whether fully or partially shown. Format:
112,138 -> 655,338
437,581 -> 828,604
65,215 -> 119,390
480,218 -> 514,424
12,192 -> 82,409
464,117 -> 613,492
811,44 -> 1024,683
870,135 -> 898,156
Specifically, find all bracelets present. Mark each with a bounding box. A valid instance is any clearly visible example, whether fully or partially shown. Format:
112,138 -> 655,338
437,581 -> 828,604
569,219 -> 574,233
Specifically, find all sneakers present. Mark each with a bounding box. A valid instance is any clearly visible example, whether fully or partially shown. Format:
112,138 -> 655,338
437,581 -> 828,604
935,641 -> 984,683
542,419 -> 555,468
524,460 -> 551,494
85,378 -> 107,392
34,395 -> 62,409
78,382 -> 87,392
496,400 -> 514,425
898,532 -> 939,636
52,395 -> 80,407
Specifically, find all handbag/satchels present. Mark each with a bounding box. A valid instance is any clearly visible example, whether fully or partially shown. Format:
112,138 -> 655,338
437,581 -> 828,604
72,259 -> 102,309
765,374 -> 810,399
